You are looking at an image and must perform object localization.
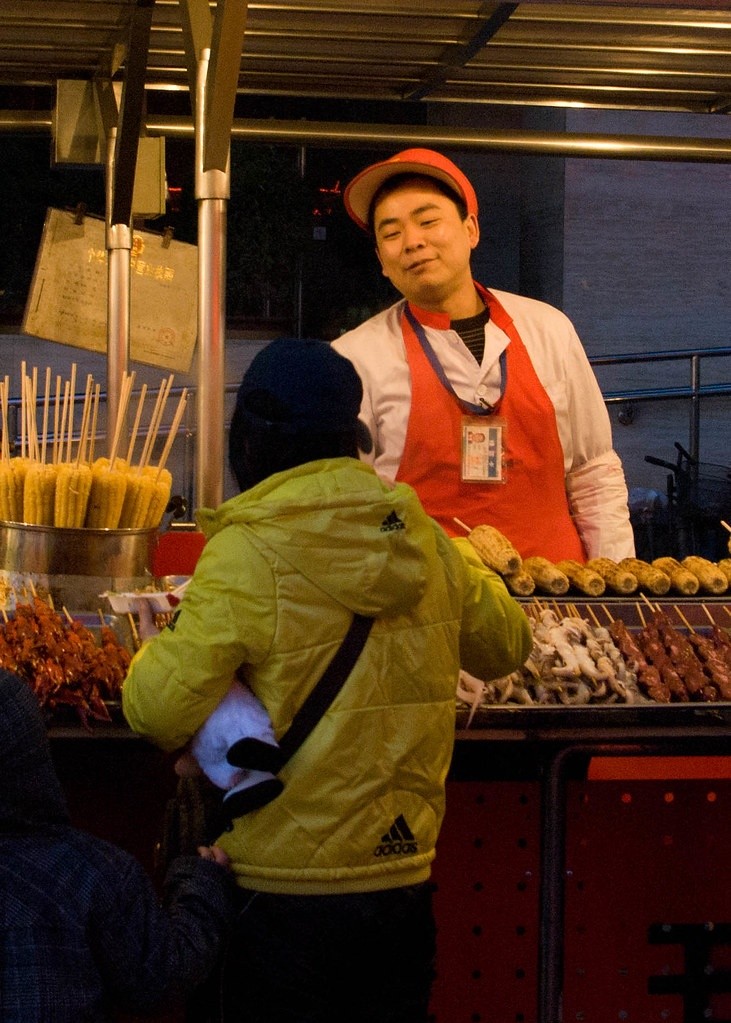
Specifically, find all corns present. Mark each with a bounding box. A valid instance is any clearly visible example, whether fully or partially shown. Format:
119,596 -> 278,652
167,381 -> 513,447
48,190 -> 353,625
0,455 -> 173,529
465,524 -> 731,598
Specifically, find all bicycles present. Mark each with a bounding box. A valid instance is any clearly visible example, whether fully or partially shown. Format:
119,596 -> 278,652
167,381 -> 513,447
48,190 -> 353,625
629,443 -> 731,560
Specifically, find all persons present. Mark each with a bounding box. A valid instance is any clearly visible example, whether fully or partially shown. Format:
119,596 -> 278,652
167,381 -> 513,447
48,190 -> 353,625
0,666 -> 241,1023
191,675 -> 284,818
123,336 -> 534,1023
328,146 -> 637,562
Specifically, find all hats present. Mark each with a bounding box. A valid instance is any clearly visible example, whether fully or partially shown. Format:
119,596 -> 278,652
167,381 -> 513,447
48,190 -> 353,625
0,668 -> 65,808
343,148 -> 479,230
236,338 -> 374,452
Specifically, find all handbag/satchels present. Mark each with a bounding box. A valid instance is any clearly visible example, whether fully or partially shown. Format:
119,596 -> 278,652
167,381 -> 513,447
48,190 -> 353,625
152,775 -> 223,877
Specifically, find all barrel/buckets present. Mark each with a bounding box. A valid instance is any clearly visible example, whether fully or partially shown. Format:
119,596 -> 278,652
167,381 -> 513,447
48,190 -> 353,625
0,520 -> 156,588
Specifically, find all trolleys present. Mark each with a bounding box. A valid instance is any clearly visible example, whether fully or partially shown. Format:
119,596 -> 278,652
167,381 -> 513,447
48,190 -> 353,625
0,0 -> 731,1023
